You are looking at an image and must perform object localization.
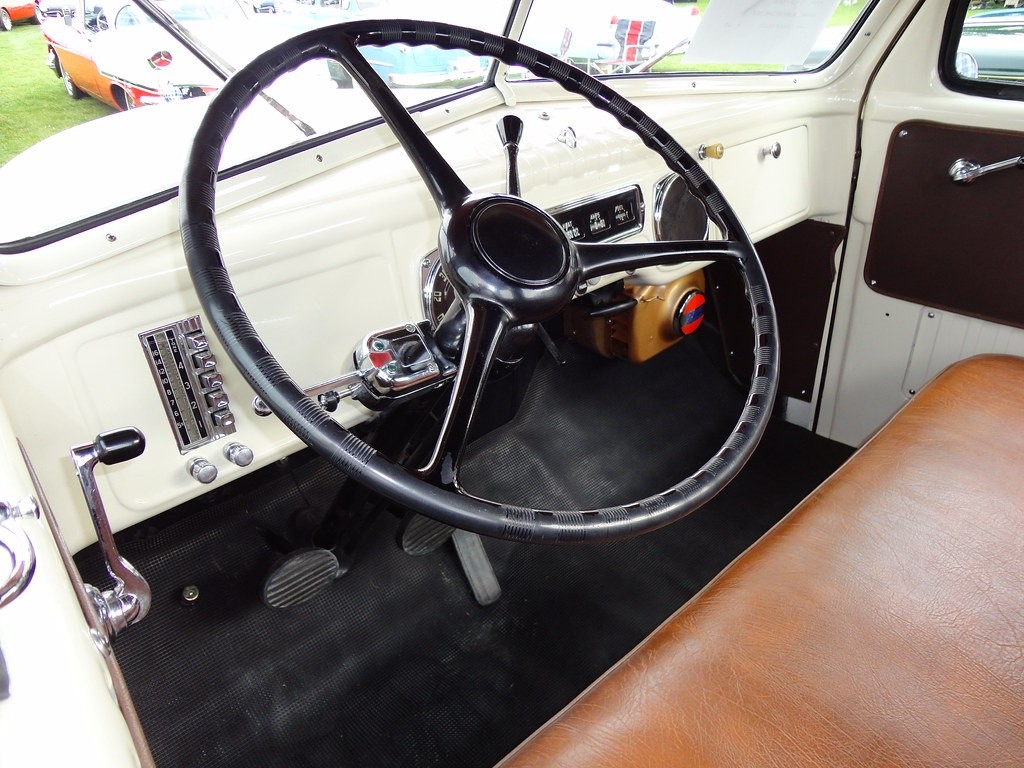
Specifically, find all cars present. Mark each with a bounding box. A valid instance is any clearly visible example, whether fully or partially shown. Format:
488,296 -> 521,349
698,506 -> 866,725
0,0 -> 1024,112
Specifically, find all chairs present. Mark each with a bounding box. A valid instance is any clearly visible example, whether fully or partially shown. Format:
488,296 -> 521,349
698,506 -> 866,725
490,354 -> 1024,767
